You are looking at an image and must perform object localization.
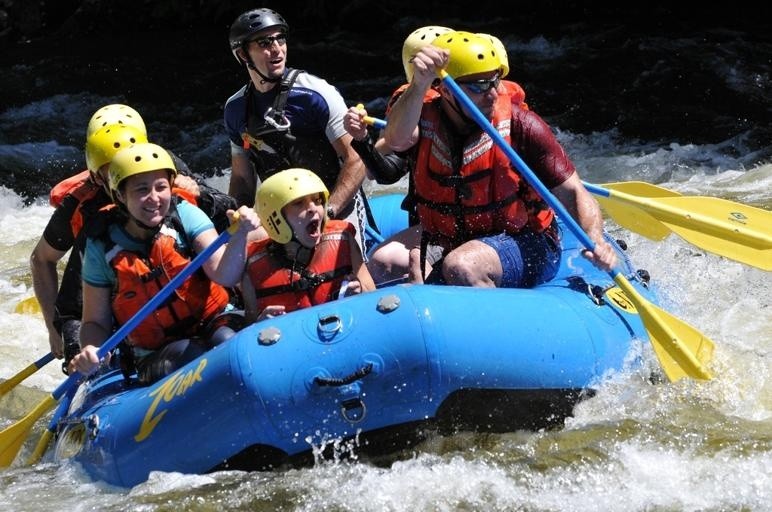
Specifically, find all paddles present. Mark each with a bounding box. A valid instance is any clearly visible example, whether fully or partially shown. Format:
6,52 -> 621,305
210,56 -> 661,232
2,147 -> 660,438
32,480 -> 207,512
0,213 -> 245,477
14,283 -> 39,322
356,103 -> 682,241
437,69 -> 715,382
577,179 -> 772,274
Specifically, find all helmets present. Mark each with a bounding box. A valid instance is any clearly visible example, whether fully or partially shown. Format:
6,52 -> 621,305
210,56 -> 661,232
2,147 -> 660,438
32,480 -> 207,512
108,144 -> 177,202
82,125 -> 150,170
253,168 -> 330,244
227,7 -> 291,64
427,31 -> 502,81
86,102 -> 146,135
475,32 -> 509,80
401,26 -> 454,84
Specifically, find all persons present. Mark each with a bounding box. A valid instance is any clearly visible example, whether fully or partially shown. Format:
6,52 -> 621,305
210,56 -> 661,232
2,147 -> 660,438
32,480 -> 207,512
222,8 -> 368,260
29,104 -> 377,387
344,25 -> 618,289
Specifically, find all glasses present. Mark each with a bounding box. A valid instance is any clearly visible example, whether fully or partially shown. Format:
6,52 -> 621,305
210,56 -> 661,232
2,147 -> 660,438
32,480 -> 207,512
240,33 -> 288,47
455,76 -> 502,93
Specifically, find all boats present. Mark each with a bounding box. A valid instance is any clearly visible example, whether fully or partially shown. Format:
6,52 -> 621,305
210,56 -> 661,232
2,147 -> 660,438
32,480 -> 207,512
57,195 -> 658,490
